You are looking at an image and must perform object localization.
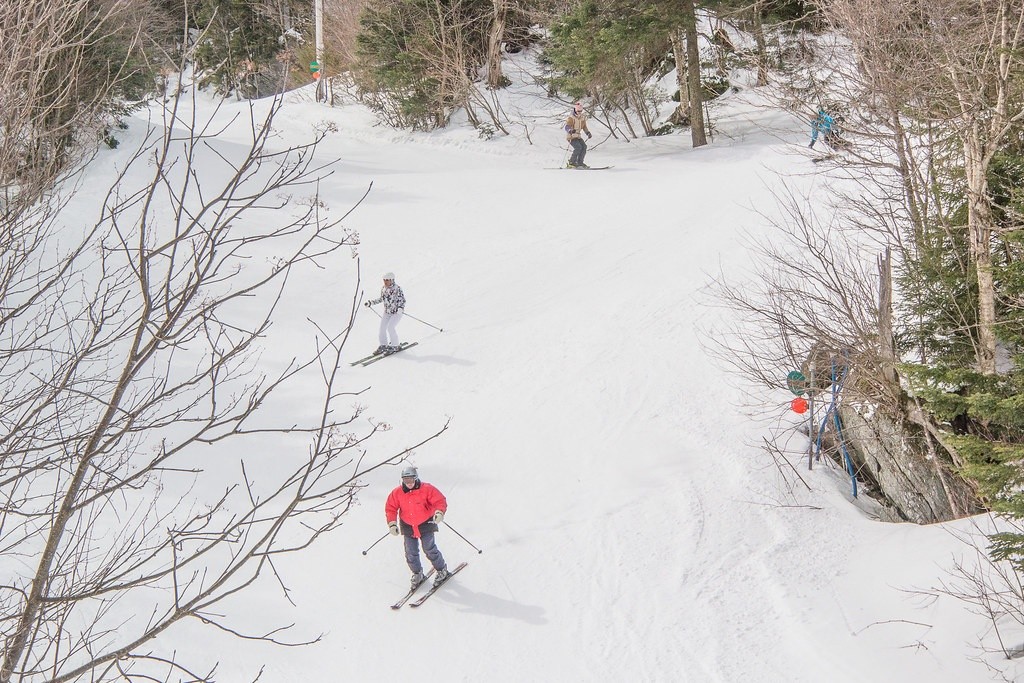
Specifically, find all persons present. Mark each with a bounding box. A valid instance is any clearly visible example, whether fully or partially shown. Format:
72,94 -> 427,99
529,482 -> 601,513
364,273 -> 406,353
565,103 -> 592,169
386,467 -> 448,587
808,112 -> 852,153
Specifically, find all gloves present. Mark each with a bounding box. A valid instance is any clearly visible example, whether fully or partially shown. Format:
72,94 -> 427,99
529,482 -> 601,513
397,308 -> 402,314
571,129 -> 576,133
391,526 -> 400,536
433,514 -> 443,524
365,301 -> 370,307
587,132 -> 592,139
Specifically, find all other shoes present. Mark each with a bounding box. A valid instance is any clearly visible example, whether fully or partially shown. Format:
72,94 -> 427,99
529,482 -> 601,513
385,346 -> 401,355
579,164 -> 586,168
374,345 -> 385,355
568,161 -> 576,167
411,571 -> 423,585
434,568 -> 447,581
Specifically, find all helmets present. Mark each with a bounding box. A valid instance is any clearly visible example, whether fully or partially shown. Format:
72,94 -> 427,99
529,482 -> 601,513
402,468 -> 416,478
383,273 -> 395,280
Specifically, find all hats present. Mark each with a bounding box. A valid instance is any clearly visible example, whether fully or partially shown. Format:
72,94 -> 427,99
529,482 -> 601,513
575,102 -> 583,112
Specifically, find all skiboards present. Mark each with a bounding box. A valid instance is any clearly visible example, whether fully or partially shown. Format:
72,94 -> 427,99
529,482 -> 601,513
390,562 -> 468,609
349,341 -> 419,366
543,166 -> 615,170
812,147 -> 866,163
